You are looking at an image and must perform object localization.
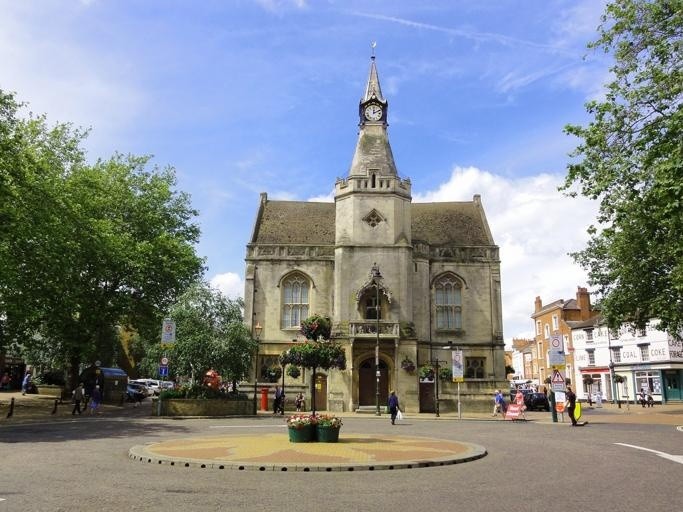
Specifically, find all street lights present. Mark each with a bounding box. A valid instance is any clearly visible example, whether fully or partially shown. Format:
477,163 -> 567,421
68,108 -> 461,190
443,346 -> 462,418
373,265 -> 385,415
252,321 -> 262,415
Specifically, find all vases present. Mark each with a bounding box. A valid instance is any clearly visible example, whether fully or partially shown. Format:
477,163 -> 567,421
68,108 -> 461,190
286,424 -> 340,443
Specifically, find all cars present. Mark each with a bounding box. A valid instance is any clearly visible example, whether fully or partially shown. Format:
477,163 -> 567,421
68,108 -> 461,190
125,378 -> 177,402
510,383 -> 550,412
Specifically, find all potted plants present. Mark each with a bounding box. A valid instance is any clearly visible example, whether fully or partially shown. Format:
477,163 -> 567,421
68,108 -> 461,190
416,363 -> 435,384
266,361 -> 281,383
401,354 -> 415,372
288,362 -> 301,377
439,366 -> 455,384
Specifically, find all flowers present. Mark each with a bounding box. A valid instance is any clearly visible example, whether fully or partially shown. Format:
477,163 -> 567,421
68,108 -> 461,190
287,412 -> 343,430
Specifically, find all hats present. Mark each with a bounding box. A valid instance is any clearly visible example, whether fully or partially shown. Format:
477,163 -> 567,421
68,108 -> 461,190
391,390 -> 395,394
495,390 -> 499,393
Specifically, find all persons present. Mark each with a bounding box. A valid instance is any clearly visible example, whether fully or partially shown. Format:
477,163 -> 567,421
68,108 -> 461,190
1,370 -> 146,417
273,384 -> 305,415
491,383 -> 653,425
388,390 -> 399,424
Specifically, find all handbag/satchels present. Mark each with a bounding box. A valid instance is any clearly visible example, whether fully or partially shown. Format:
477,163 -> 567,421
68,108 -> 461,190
496,404 -> 500,408
396,411 -> 403,419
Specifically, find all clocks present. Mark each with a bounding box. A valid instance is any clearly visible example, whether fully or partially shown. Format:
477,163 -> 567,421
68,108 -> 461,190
364,105 -> 383,122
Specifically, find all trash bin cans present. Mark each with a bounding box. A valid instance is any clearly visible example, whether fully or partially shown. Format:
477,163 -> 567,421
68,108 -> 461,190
158,399 -> 168,416
